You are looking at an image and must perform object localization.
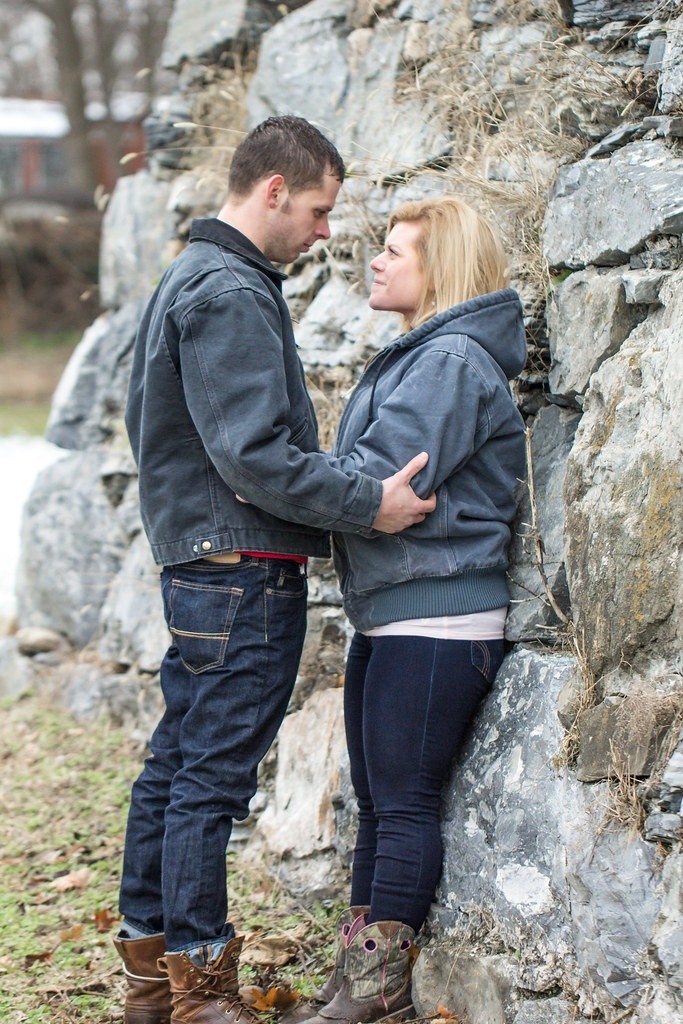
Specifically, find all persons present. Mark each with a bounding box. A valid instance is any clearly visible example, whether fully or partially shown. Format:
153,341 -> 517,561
235,200 -> 528,1023
118,117 -> 437,1023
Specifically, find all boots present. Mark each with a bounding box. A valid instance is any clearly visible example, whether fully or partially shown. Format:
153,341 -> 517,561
113,929 -> 174,1024
279,905 -> 371,1024
157,935 -> 270,1024
298,914 -> 416,1024
